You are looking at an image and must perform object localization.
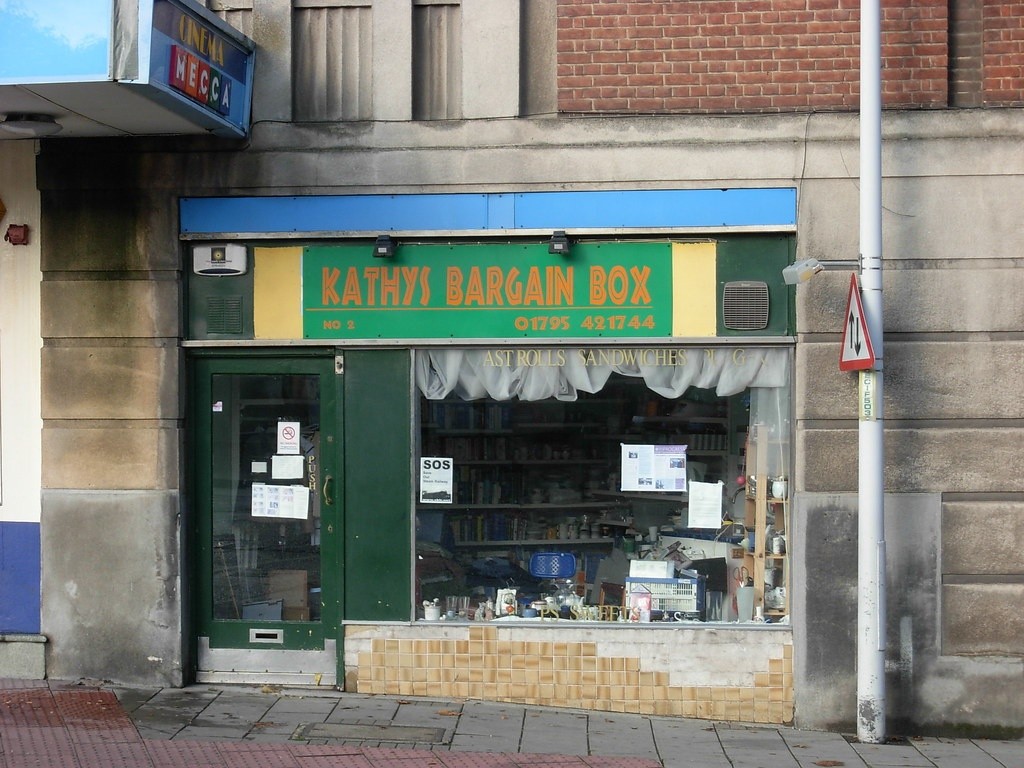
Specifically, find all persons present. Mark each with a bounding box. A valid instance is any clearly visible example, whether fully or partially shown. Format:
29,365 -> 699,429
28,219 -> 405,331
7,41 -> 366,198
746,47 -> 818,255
642,478 -> 650,485
656,480 -> 664,489
631,452 -> 636,457
671,458 -> 684,468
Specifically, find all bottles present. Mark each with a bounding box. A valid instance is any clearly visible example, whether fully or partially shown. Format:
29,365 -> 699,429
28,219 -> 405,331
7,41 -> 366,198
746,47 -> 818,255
424,606 -> 441,620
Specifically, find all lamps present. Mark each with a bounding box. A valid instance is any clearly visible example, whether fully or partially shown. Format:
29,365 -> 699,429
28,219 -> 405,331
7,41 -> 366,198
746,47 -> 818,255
373,235 -> 398,257
549,231 -> 572,253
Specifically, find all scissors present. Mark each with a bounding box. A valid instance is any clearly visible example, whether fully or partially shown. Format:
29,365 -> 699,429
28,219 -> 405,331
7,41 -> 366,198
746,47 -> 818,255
732,566 -> 749,586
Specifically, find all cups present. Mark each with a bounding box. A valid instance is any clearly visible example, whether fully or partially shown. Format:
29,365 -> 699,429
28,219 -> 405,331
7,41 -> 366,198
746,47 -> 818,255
649,527 -> 658,542
458,596 -> 471,621
559,523 -> 569,539
446,596 -> 457,621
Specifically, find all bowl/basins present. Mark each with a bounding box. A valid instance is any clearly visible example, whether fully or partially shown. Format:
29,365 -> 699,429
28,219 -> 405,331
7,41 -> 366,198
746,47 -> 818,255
523,609 -> 537,618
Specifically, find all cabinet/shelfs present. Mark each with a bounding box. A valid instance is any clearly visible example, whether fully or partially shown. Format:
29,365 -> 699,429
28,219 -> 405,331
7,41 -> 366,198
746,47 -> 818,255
414,374 -> 791,626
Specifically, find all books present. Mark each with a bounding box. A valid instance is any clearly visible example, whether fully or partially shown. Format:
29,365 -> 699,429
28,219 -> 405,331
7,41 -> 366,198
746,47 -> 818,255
421,398 -> 527,541
